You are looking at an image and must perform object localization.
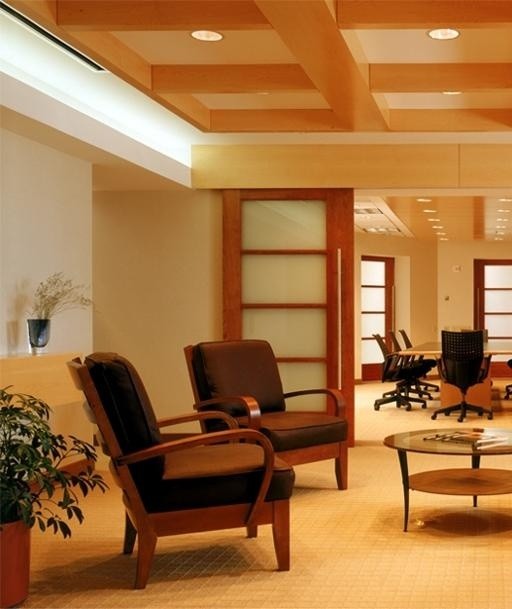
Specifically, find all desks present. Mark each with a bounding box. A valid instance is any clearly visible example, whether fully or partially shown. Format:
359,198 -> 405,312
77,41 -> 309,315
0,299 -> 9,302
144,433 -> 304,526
398,339 -> 512,414
382,427 -> 512,533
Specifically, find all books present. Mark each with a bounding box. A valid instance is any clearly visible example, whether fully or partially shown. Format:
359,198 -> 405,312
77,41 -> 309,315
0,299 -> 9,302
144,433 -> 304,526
420,428 -> 510,451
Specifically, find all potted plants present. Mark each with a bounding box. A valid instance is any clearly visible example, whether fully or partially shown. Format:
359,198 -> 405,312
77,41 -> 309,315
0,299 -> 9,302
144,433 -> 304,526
27,271 -> 97,353
0,383 -> 111,609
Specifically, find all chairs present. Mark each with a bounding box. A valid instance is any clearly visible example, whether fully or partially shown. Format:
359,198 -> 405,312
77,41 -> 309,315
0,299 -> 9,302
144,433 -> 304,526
397,328 -> 439,393
66,351 -> 296,589
383,331 -> 433,401
461,329 -> 488,342
504,359 -> 512,399
373,333 -> 427,411
431,330 -> 493,422
184,338 -> 353,490
